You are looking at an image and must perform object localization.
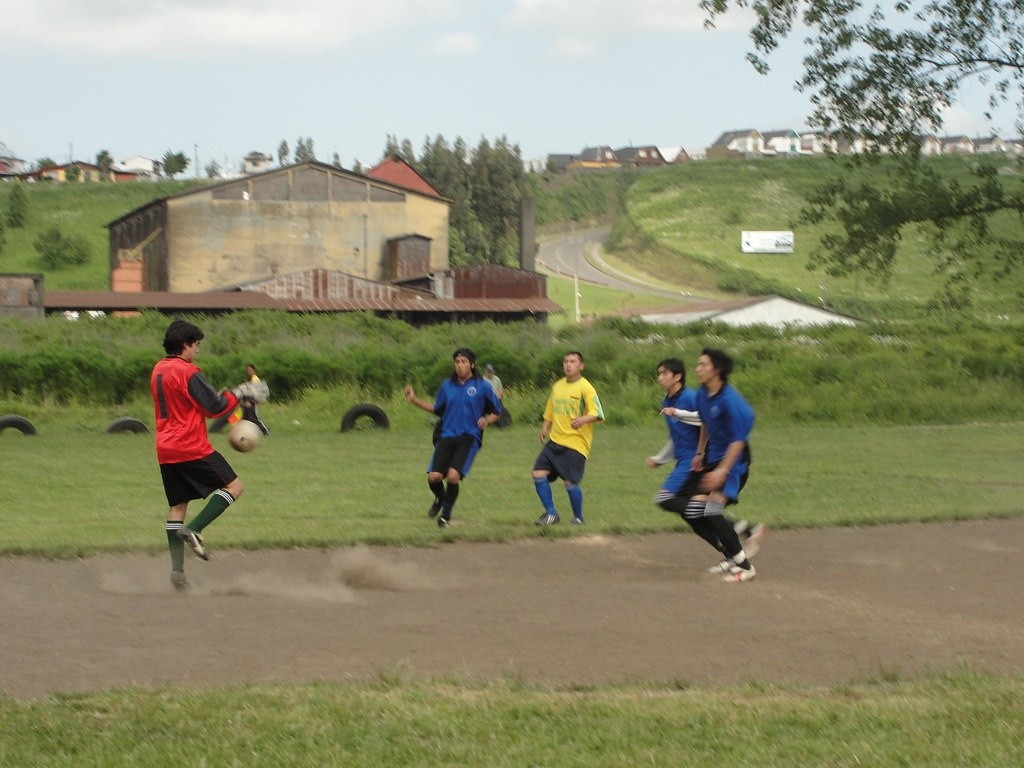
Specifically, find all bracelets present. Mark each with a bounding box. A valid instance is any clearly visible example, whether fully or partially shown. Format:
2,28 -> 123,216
696,451 -> 706,456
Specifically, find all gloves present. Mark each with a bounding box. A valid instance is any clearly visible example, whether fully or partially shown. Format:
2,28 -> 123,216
231,380 -> 270,403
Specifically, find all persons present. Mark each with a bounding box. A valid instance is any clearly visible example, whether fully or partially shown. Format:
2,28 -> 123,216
483,364 -> 505,405
673,347 -> 758,585
403,348 -> 502,529
531,350 -> 605,527
645,358 -> 767,562
239,362 -> 270,439
150,320 -> 271,596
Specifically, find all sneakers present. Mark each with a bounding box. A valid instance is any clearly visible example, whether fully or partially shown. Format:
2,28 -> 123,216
708,559 -> 757,584
428,495 -> 451,529
568,517 -> 586,526
169,570 -> 190,595
535,511 -> 561,526
176,526 -> 209,563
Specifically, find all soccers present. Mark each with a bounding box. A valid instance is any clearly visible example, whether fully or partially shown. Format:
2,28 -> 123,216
229,419 -> 262,451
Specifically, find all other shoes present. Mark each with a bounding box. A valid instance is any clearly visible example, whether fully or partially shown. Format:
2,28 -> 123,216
741,522 -> 766,559
